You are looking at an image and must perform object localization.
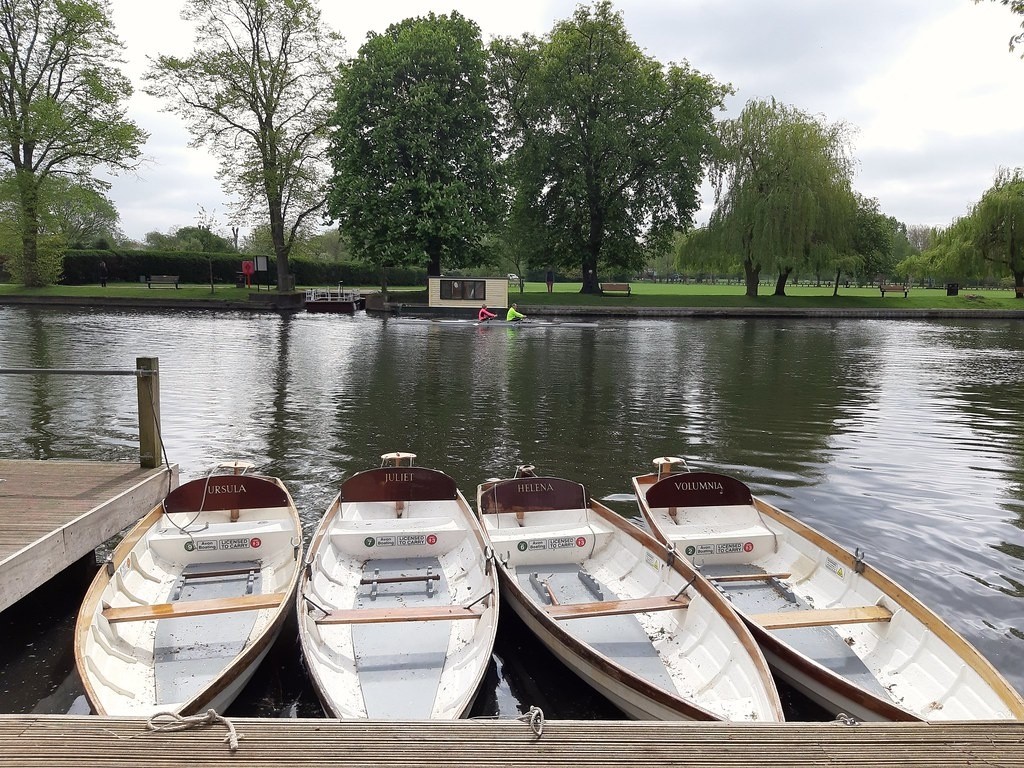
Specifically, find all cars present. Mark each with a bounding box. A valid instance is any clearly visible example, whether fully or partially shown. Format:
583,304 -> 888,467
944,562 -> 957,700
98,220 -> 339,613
507,273 -> 519,280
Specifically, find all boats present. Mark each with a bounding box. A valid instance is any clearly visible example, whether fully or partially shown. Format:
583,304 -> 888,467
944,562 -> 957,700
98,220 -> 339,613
74,459 -> 303,717
633,455 -> 1024,722
477,462 -> 785,721
432,319 -> 598,328
296,451 -> 500,719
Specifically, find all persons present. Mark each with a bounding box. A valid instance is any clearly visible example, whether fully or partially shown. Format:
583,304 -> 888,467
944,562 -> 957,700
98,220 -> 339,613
478,304 -> 497,321
96,257 -> 107,288
506,303 -> 527,321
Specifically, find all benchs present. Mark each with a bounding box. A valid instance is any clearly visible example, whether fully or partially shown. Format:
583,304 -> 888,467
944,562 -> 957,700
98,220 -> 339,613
147,275 -> 180,289
600,283 -> 631,296
880,284 -> 909,298
